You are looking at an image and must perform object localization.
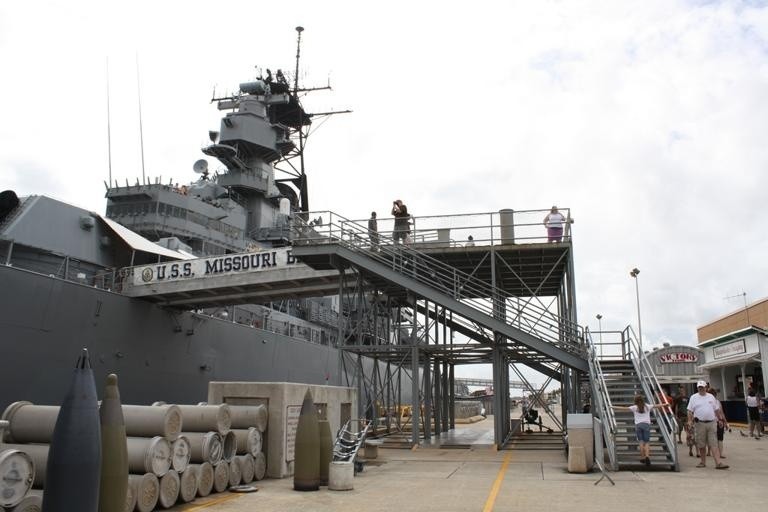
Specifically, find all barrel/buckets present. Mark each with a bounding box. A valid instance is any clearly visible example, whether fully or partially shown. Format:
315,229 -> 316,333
328,461 -> 354,491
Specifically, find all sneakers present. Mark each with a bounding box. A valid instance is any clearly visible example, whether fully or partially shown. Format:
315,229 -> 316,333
646,457 -> 650,465
640,459 -> 645,463
715,462 -> 729,469
696,463 -> 705,467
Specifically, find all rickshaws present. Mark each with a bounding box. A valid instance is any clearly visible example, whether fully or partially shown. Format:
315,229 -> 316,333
521,406 -> 542,432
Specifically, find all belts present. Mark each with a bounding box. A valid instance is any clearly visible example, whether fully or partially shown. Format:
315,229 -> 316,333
700,421 -> 712,423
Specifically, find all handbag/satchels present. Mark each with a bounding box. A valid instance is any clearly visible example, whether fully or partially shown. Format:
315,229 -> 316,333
719,420 -> 724,428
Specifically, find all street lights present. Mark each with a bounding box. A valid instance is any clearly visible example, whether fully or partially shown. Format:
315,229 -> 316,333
630,267 -> 644,379
596,314 -> 603,361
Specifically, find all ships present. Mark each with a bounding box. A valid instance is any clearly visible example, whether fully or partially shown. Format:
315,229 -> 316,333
0,24 -> 465,421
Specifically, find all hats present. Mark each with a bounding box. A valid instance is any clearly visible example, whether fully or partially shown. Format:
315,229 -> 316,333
696,381 -> 706,388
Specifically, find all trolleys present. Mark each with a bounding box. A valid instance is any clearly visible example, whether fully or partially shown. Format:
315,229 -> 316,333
333,418 -> 374,476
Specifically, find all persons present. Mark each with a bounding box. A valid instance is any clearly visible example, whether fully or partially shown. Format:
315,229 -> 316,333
464,235 -> 474,247
391,199 -> 412,245
611,393 -> 671,466
367,212 -> 379,252
543,206 -> 566,243
521,396 -> 527,415
657,382 -> 768,469
